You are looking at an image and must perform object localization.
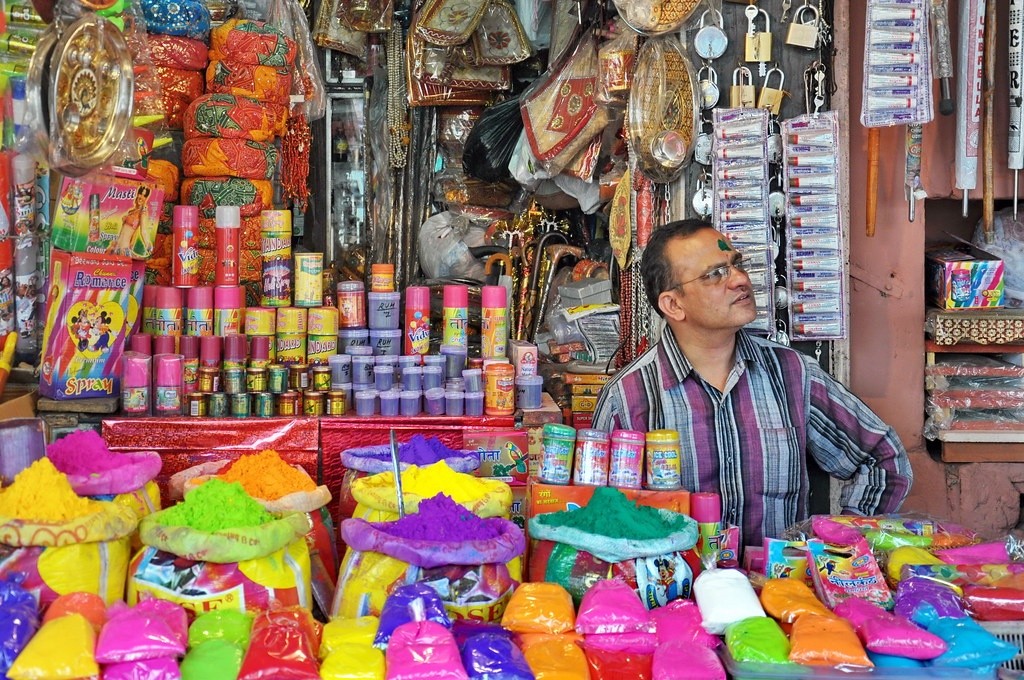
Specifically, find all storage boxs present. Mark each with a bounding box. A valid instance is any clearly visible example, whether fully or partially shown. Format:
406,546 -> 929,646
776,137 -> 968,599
547,339 -> 586,355
570,384 -> 605,395
102,415 -> 320,453
49,423 -> 102,448
925,230 -> 1005,312
561,372 -> 611,384
571,396 -> 599,412
93,126 -> 154,182
37,413 -> 79,428
0,418 -> 46,487
518,391 -> 563,482
131,450 -> 319,515
319,423 -> 529,490
0,382 -> 40,420
40,246 -> 148,401
48,167 -> 166,261
553,353 -> 571,363
507,338 -> 539,377
924,306 -> 1024,346
558,278 -> 612,307
326,486 -> 531,584
573,412 -> 593,424
523,476 -> 691,582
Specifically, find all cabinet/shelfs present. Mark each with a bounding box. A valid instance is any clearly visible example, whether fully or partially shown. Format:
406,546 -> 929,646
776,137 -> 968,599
925,340 -> 1024,430
311,92 -> 367,271
314,0 -> 364,87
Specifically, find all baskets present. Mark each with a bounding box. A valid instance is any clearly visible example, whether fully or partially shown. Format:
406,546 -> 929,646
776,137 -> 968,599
980,620 -> 1024,680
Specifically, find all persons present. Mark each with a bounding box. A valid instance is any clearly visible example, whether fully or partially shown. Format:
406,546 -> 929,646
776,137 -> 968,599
105,185 -> 153,257
591,219 -> 913,570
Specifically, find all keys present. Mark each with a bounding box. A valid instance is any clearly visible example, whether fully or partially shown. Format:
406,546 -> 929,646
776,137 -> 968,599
813,70 -> 825,119
768,111 -> 774,135
775,162 -> 783,186
780,0 -> 791,23
699,173 -> 707,201
815,341 -> 822,362
774,262 -> 778,283
745,5 -> 759,37
775,214 -> 783,246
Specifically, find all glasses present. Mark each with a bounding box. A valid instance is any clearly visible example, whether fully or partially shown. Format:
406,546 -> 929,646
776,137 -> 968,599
666,257 -> 751,292
